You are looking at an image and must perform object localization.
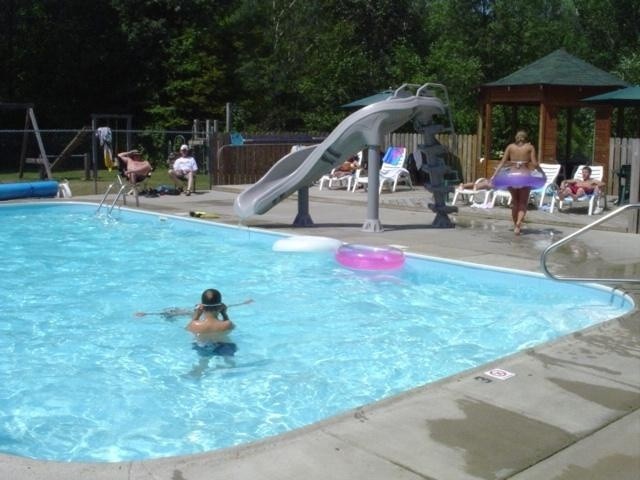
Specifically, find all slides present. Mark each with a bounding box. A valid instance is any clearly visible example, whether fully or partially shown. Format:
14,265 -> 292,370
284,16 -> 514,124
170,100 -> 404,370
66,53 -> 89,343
236,95 -> 444,218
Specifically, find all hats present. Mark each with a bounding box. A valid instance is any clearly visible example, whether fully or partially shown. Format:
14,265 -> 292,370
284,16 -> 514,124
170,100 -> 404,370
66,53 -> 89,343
181,144 -> 189,151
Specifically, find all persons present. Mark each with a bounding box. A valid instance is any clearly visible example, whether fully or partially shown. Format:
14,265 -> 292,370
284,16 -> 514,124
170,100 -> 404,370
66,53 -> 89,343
186,288 -> 237,374
557,166 -> 606,201
328,154 -> 361,178
117,149 -> 153,188
168,144 -> 198,196
489,129 -> 546,236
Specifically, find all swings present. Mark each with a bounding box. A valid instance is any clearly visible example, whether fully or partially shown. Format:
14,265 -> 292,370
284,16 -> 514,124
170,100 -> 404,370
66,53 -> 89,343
107,119 -> 119,167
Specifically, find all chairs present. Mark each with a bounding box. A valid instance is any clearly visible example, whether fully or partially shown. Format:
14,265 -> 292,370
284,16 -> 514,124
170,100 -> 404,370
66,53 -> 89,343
320,147 -> 413,193
119,167 -> 196,195
452,163 -> 608,216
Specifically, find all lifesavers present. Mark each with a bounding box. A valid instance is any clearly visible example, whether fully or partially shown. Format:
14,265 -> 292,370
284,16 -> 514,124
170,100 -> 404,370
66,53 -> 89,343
335,244 -> 405,271
272,236 -> 342,255
492,167 -> 547,191
